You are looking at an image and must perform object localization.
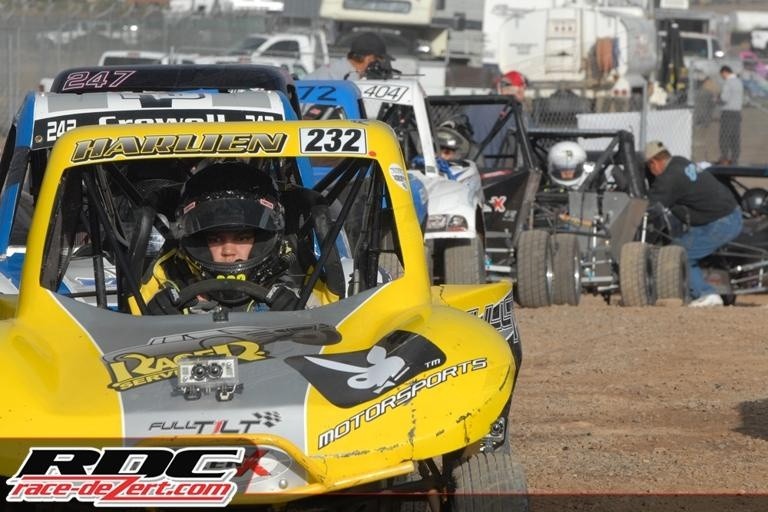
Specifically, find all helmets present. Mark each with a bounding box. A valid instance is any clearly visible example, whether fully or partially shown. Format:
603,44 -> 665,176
172,161 -> 288,305
435,120 -> 471,160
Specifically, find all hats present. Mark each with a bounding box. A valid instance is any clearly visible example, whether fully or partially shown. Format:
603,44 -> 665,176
350,32 -> 396,61
495,71 -> 525,87
641,140 -> 666,164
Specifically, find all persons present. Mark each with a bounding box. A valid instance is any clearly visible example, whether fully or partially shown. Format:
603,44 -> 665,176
641,139 -> 743,308
432,126 -> 468,166
496,70 -> 531,101
718,63 -> 748,167
302,33 -> 398,80
120,226 -> 337,320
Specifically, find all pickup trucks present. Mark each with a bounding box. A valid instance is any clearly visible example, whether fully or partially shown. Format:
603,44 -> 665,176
215,27 -> 343,73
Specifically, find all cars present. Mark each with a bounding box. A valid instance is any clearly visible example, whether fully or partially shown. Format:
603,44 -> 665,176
657,28 -> 726,68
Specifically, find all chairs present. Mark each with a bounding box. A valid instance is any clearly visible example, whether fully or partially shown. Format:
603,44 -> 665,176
123,160 -> 346,303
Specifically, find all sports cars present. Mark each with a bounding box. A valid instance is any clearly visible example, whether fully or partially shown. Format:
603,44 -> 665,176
0,66 -> 767,512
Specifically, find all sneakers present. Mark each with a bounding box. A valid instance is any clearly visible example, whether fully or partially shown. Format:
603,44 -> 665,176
686,293 -> 725,309
